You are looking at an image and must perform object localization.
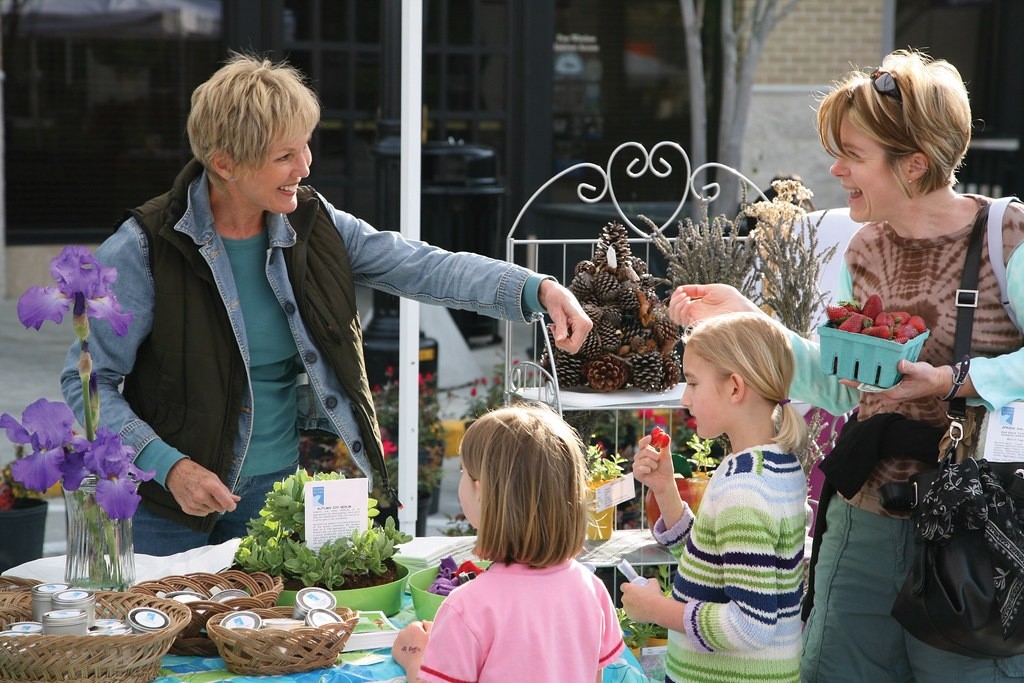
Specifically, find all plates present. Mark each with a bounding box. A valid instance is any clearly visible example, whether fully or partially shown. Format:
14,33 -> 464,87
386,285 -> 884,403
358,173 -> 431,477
2,555 -> 66,584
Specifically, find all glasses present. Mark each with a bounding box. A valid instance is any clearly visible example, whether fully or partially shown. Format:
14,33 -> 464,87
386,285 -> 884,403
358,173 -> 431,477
869,69 -> 906,102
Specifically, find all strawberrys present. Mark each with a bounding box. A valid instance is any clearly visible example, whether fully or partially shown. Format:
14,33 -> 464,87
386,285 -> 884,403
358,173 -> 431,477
827,294 -> 926,343
651,426 -> 670,448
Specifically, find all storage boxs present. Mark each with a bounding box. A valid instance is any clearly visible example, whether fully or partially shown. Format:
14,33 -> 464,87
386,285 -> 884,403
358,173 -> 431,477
816,318 -> 931,388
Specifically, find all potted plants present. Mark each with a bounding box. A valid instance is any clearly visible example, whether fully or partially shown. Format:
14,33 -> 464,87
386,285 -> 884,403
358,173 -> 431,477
233,464 -> 413,622
646,432 -> 721,552
645,564 -> 674,647
579,444 -> 629,541
613,607 -> 646,658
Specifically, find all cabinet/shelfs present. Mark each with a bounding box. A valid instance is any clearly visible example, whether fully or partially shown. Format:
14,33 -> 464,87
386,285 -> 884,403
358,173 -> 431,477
501,142 -> 773,609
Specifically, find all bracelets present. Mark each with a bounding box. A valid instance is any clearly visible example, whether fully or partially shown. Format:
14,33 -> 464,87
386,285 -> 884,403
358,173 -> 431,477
936,354 -> 970,402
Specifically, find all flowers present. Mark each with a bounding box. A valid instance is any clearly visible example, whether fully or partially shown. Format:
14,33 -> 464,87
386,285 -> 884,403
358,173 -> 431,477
1,444 -> 32,513
1,246 -> 159,528
311,359 -> 524,489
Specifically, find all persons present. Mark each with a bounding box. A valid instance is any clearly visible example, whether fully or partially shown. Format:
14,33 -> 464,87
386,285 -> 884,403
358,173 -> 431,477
620,312 -> 808,683
60,55 -> 593,557
391,404 -> 626,683
669,51 -> 1024,683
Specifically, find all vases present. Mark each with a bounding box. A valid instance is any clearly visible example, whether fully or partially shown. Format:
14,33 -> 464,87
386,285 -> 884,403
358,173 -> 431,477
417,480 -> 442,516
57,472 -> 137,593
0,497 -> 48,579
377,493 -> 433,537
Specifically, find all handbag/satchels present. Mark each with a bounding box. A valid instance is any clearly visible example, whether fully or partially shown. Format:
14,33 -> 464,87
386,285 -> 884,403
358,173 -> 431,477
891,457 -> 1024,658
